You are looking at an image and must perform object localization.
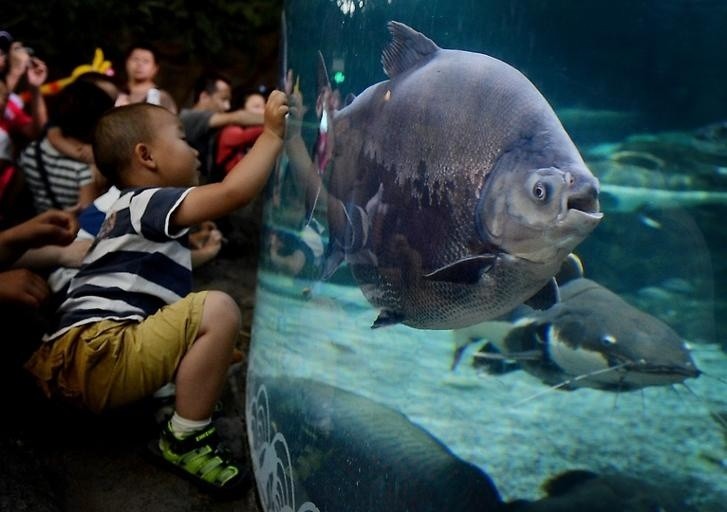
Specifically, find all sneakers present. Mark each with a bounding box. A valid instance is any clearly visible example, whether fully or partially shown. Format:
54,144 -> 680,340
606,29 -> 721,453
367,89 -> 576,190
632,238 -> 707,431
156,419 -> 253,502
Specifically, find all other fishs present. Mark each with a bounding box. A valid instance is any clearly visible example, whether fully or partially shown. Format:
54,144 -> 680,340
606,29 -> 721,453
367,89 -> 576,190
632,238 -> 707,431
448,251 -> 727,415
294,19 -> 605,331
580,120 -> 726,230
252,373 -> 506,512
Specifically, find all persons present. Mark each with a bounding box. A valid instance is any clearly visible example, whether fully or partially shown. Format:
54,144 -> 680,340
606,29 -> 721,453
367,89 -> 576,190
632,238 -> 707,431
179,75 -> 265,181
2,31 -> 177,309
269,71 -> 372,277
28,90 -> 290,495
